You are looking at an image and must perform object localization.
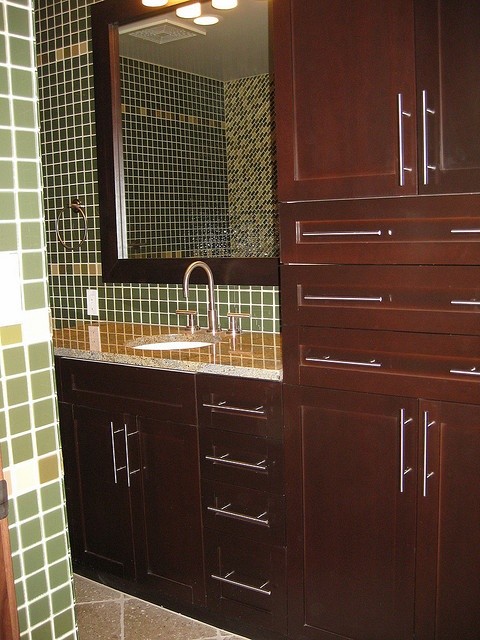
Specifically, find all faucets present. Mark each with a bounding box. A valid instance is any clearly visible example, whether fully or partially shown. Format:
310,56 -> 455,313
182,260 -> 221,333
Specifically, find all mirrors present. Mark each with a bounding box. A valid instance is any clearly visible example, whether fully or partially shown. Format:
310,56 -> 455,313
89,1 -> 276,285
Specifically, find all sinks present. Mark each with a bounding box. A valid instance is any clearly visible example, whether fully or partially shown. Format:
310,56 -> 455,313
127,333 -> 221,351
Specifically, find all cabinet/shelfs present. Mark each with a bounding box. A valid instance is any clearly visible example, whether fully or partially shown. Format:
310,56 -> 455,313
195,372 -> 284,640
54,353 -> 198,638
269,0 -> 480,639
